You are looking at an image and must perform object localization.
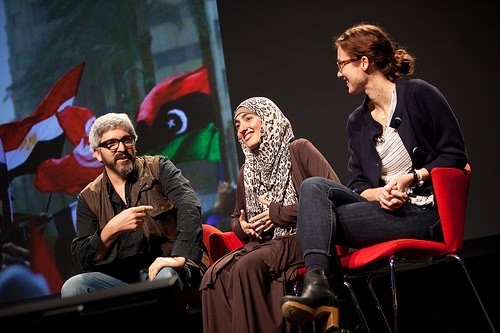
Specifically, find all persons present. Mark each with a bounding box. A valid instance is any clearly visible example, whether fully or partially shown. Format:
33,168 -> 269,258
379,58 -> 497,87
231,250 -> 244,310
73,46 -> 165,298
199,97 -> 341,333
280,25 -> 467,330
61,112 -> 210,299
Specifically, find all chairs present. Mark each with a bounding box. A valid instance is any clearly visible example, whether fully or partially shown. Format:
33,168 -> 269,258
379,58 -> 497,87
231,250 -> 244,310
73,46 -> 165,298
202,164 -> 497,333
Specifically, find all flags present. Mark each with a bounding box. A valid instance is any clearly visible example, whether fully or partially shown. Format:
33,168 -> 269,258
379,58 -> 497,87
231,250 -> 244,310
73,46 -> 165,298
0,59 -> 225,198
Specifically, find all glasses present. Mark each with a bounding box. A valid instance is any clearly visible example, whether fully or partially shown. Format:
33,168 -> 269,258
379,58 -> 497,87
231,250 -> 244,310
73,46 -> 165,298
336,59 -> 357,73
98,135 -> 135,150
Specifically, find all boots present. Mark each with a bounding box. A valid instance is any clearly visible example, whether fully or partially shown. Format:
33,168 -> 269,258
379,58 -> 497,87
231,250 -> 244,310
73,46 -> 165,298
280,267 -> 340,333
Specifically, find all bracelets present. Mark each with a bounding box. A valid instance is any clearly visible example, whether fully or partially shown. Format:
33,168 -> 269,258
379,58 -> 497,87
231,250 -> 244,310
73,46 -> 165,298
407,169 -> 423,188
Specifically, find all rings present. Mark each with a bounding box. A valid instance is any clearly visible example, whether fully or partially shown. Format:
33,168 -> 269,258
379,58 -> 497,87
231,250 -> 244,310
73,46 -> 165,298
260,219 -> 266,225
